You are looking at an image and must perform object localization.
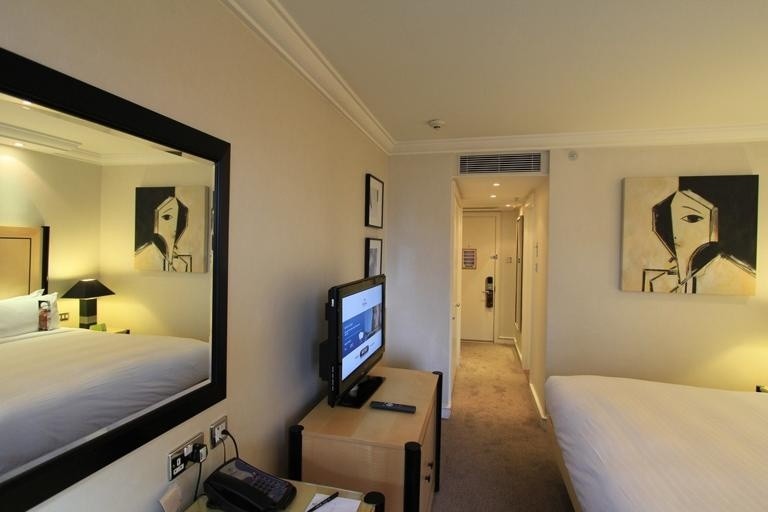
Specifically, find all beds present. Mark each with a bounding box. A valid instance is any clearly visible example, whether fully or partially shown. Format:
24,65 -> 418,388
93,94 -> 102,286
1,226 -> 210,475
546,376 -> 768,511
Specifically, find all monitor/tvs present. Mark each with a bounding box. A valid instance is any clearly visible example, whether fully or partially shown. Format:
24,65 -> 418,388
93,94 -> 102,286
318,273 -> 389,411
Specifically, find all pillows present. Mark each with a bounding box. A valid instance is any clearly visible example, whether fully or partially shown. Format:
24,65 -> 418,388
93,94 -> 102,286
1,289 -> 44,339
39,291 -> 59,331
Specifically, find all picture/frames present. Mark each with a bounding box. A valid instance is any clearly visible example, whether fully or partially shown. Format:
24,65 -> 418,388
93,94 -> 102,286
365,173 -> 384,229
364,237 -> 383,278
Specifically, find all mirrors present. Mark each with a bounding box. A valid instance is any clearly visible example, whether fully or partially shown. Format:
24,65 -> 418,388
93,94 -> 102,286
1,47 -> 229,512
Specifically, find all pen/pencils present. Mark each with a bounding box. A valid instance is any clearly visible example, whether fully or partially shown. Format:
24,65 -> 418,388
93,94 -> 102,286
307,492 -> 339,512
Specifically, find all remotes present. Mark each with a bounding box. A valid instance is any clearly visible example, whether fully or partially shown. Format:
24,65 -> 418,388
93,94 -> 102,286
370,398 -> 417,415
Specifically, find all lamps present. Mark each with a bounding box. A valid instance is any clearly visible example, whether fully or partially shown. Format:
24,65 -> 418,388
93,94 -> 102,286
60,278 -> 117,329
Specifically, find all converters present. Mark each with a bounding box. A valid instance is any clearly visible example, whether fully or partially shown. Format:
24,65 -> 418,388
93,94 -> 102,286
189,443 -> 208,462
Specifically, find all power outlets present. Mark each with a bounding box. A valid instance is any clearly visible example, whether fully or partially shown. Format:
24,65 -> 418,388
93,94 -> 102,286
210,415 -> 228,449
169,431 -> 204,481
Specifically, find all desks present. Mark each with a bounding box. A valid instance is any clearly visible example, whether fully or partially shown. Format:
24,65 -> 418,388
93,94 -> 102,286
290,366 -> 443,512
184,476 -> 385,512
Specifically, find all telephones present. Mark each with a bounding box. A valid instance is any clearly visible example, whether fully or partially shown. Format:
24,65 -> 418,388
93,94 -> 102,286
204,458 -> 297,512
